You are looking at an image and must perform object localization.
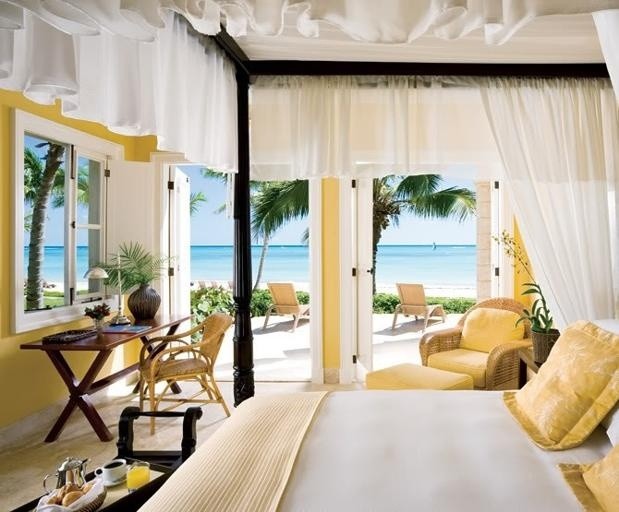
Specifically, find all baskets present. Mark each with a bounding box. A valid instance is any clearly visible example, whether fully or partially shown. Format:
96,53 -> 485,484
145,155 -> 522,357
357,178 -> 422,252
32,485 -> 107,512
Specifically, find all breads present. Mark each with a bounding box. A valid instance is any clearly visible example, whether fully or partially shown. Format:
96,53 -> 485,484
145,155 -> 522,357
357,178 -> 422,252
48,482 -> 95,506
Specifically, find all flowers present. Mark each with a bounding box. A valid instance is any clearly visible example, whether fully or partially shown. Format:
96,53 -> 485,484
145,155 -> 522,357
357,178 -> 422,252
85,303 -> 111,320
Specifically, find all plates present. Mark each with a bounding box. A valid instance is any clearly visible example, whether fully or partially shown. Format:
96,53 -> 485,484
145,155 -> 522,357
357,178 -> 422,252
99,465 -> 131,487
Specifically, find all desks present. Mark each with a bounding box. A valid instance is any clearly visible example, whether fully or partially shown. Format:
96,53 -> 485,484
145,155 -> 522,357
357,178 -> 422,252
19,314 -> 193,443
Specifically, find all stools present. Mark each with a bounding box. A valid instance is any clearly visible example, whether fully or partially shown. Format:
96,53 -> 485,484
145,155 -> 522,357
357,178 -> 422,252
366,364 -> 473,389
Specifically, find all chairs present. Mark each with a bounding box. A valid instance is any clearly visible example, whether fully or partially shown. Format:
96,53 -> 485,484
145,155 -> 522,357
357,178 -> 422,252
419,298 -> 532,390
391,282 -> 446,332
139,313 -> 234,435
262,282 -> 310,332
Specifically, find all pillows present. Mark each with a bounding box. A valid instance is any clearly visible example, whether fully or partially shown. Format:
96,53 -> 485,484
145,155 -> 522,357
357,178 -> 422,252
558,443 -> 619,512
502,318 -> 619,451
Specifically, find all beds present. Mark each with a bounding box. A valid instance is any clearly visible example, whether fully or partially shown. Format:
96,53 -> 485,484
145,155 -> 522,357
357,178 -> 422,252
136,320 -> 619,512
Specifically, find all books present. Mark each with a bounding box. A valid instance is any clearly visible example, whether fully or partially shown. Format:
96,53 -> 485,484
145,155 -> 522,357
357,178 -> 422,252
102,325 -> 152,333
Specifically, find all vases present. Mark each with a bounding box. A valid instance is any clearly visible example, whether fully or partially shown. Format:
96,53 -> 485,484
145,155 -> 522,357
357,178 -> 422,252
94,317 -> 106,340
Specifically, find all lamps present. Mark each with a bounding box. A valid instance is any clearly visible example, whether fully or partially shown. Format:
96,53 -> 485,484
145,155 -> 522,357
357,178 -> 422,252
84,251 -> 131,326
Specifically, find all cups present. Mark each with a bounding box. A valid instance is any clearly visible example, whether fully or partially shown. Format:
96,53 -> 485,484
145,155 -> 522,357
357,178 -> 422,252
94,459 -> 127,482
127,460 -> 150,493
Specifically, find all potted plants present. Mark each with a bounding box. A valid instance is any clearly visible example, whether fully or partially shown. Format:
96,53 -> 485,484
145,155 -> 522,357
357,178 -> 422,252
89,241 -> 177,319
489,229 -> 560,364
